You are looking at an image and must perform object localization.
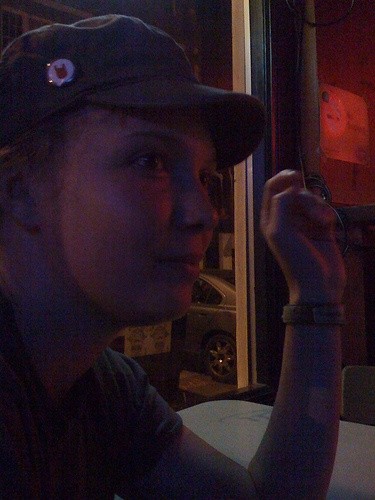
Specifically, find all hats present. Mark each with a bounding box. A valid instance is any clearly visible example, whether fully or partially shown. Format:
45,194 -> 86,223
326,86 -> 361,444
0,14 -> 266,173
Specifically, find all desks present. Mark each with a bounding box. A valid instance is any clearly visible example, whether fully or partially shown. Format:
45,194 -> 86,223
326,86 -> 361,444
176,400 -> 375,500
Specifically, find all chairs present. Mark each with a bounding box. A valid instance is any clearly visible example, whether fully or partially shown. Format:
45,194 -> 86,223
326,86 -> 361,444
341,365 -> 375,425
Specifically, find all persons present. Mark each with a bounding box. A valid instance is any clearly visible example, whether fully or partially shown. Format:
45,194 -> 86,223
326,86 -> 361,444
0,14 -> 345,500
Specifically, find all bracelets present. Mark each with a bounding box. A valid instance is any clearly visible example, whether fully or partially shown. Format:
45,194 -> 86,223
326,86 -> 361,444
282,302 -> 345,326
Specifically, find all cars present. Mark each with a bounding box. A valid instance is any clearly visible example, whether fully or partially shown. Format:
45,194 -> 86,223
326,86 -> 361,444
173,273 -> 237,382
